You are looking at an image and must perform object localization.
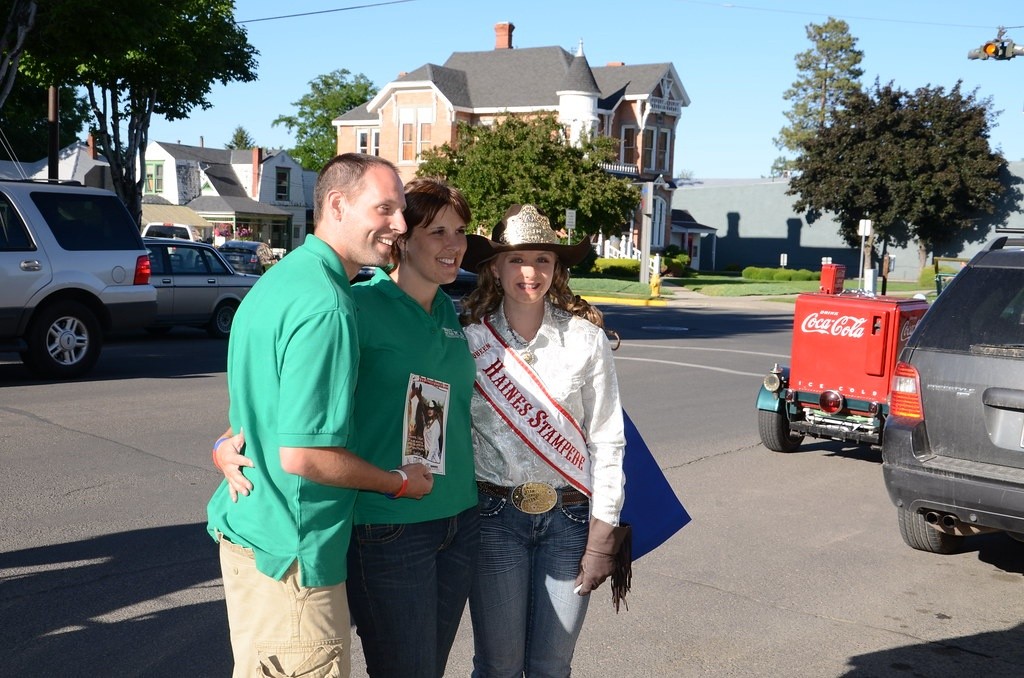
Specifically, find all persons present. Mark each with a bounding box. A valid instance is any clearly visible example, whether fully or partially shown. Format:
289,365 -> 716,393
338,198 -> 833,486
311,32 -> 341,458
211,179 -> 484,678
205,152 -> 435,676
458,203 -> 628,678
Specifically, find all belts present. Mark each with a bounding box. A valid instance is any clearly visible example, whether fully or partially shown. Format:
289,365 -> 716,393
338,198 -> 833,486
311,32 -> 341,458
475,482 -> 588,513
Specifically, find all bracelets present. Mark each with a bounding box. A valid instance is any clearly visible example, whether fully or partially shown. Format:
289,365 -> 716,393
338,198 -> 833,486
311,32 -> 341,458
211,437 -> 230,472
384,469 -> 408,499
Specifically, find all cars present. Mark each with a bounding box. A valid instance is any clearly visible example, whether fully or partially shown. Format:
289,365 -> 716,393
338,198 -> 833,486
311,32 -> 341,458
757,293 -> 930,461
440,268 -> 489,318
103,237 -> 262,340
219,240 -> 280,279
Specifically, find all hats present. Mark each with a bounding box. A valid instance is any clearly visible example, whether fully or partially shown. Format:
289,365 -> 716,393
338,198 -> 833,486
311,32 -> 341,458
461,205 -> 590,274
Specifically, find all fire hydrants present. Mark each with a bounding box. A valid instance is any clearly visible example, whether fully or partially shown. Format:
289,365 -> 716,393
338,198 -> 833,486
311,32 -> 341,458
649,272 -> 663,300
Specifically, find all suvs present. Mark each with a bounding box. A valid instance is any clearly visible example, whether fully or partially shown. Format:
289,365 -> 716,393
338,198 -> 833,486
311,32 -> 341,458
140,221 -> 213,272
0,177 -> 158,381
880,233 -> 1024,564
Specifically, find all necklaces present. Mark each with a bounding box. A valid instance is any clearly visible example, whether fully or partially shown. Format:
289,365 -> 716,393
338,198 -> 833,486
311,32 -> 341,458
503,301 -> 543,364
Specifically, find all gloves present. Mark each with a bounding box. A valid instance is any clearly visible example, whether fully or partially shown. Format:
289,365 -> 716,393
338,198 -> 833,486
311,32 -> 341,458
575,517 -> 631,597
